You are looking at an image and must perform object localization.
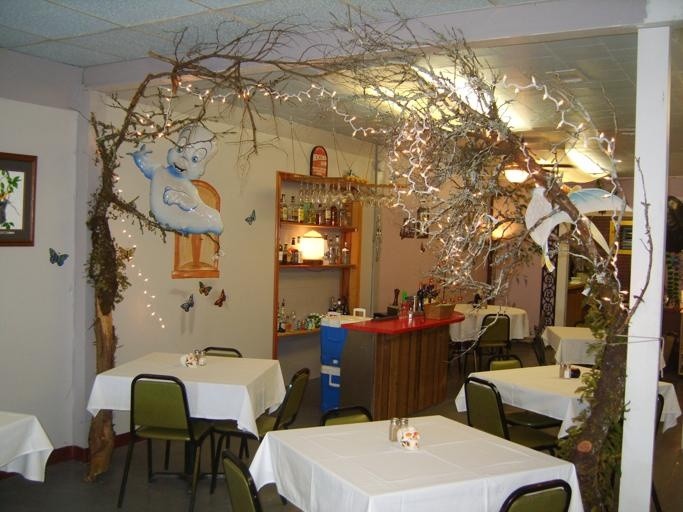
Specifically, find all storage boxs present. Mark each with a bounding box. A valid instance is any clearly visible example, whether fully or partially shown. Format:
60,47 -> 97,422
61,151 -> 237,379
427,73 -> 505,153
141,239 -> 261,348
319,366 -> 340,413
319,308 -> 374,366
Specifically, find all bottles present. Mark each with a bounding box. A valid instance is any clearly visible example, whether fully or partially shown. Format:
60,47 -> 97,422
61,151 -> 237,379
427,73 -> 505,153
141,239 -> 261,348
328,237 -> 333,264
287,195 -> 297,222
282,243 -> 289,264
278,302 -> 286,333
322,234 -> 329,265
279,193 -> 287,221
341,241 -> 350,264
335,299 -> 343,315
558,362 -> 563,379
325,206 -> 332,226
194,350 -> 199,365
328,296 -> 335,316
315,203 -> 324,226
338,208 -> 345,226
306,202 -> 316,225
289,312 -> 297,330
288,237 -> 297,264
296,236 -> 303,264
331,205 -> 337,226
297,201 -> 305,224
198,351 -> 206,366
416,277 -> 440,313
343,300 -> 350,315
346,208 -> 352,227
333,235 -> 341,264
279,243 -> 282,265
399,418 -> 408,429
563,363 -> 570,379
389,418 -> 400,442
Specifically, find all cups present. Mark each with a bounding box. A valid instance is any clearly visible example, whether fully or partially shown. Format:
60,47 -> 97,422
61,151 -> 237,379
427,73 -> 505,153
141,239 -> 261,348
397,427 -> 424,451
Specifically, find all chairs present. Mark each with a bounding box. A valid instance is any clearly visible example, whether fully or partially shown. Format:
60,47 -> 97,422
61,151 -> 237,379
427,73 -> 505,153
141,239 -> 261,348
498,478 -> 572,512
118,373 -> 215,512
209,367 -> 310,494
464,377 -> 560,456
489,355 -> 563,429
463,314 -> 511,371
321,406 -> 373,426
222,448 -> 264,511
663,329 -> 676,365
610,391 -> 662,512
202,347 -> 242,357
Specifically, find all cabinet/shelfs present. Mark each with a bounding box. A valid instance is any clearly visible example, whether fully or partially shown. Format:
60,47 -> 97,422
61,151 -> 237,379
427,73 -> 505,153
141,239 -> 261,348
271,170 -> 366,359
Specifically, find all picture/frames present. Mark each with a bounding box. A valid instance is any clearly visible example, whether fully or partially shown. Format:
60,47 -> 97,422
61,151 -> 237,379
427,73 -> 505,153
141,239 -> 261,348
0,152 -> 38,248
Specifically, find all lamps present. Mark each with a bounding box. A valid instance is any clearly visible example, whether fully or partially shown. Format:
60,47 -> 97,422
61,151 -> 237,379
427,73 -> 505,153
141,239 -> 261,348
504,162 -> 529,184
299,229 -> 325,265
